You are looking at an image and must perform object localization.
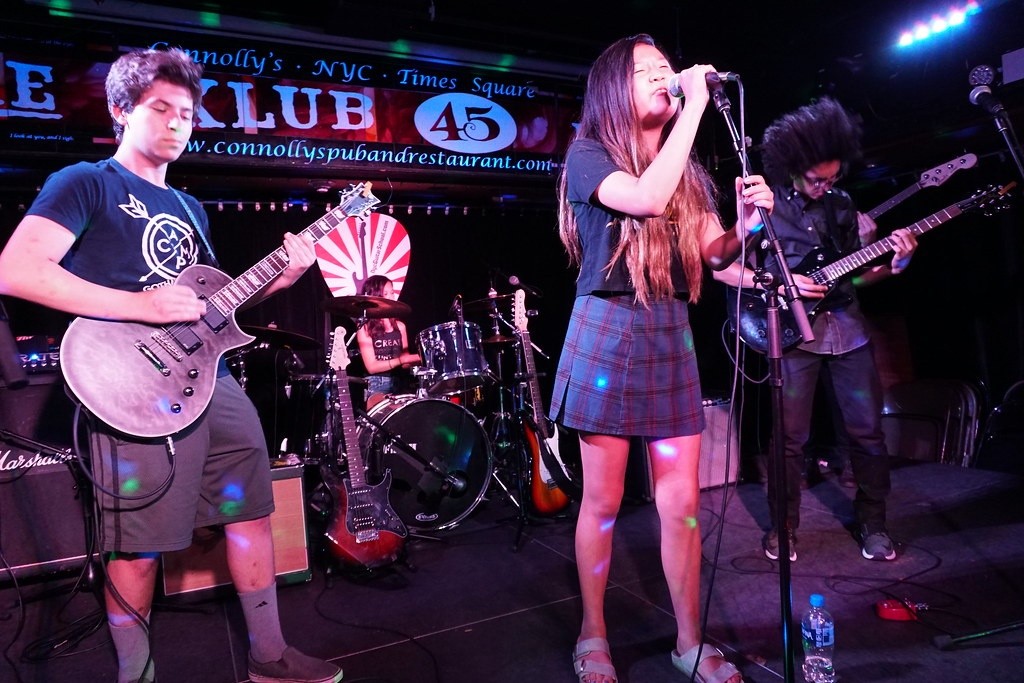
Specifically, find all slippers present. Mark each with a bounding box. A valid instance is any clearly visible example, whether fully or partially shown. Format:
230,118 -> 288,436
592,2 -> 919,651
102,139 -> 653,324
572,638 -> 619,683
671,644 -> 744,683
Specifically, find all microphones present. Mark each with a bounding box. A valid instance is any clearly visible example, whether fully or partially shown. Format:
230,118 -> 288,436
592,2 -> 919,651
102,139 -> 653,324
451,478 -> 466,495
509,276 -> 537,296
970,85 -> 1008,121
450,295 -> 460,315
667,70 -> 739,98
290,348 -> 304,369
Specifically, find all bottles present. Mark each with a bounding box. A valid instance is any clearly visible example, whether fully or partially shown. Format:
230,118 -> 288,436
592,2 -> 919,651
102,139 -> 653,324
801,593 -> 835,683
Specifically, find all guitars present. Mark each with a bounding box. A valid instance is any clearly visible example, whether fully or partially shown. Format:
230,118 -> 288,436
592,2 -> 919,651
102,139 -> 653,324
511,289 -> 578,518
866,149 -> 978,221
726,185 -> 1011,355
321,326 -> 411,585
59,182 -> 382,438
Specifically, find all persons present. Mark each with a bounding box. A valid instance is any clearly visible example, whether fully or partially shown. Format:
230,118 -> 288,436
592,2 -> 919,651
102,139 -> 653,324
713,101 -> 918,561
0,48 -> 343,683
355,275 -> 421,398
549,34 -> 773,683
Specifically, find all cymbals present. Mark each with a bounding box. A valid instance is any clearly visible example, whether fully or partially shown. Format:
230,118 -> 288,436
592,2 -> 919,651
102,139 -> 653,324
461,293 -> 514,307
238,325 -> 322,351
483,335 -> 518,345
319,295 -> 412,319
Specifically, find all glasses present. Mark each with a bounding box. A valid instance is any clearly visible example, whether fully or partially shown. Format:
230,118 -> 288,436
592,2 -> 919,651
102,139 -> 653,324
801,171 -> 843,189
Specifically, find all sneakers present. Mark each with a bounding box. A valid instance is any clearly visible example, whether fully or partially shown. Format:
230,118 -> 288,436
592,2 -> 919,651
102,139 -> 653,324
799,459 -> 820,490
857,516 -> 897,560
248,646 -> 344,683
840,462 -> 857,487
765,528 -> 797,561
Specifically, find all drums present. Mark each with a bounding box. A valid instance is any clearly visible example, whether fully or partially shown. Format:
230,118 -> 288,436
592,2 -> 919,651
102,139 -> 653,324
414,321 -> 489,396
277,373 -> 369,465
355,394 -> 493,533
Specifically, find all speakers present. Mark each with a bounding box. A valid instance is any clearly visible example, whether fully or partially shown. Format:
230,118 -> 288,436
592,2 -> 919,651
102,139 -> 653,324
161,459 -> 312,602
641,397 -> 740,500
0,373 -> 113,574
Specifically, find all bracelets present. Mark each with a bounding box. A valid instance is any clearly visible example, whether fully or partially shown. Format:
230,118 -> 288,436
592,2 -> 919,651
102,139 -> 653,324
398,357 -> 402,365
389,360 -> 393,369
